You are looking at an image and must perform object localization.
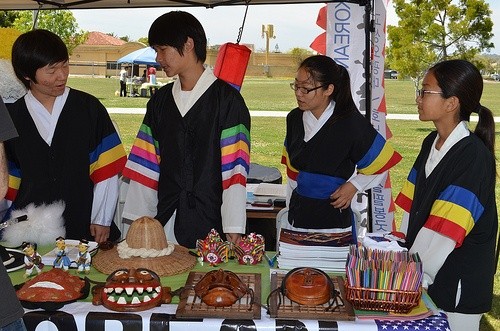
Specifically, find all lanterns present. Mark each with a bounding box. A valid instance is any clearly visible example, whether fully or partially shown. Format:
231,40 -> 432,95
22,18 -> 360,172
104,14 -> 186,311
213,42 -> 251,92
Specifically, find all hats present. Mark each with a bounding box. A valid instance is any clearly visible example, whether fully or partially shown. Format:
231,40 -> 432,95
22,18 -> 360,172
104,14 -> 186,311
90,215 -> 197,279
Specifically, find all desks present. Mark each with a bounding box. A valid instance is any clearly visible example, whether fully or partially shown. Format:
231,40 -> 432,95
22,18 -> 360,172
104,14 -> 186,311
0,202 -> 450,331
126,82 -> 167,96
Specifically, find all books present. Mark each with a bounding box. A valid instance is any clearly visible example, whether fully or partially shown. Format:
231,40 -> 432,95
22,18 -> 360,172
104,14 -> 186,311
278,227 -> 354,274
254,181 -> 286,203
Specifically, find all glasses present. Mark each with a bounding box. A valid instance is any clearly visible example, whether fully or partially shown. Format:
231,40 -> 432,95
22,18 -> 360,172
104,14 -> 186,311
290,82 -> 323,94
415,89 -> 443,98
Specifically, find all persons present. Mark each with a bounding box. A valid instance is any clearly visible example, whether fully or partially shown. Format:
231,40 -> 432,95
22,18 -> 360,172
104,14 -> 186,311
0,28 -> 128,250
51,236 -> 71,273
23,241 -> 45,278
278,53 -> 403,256
121,10 -> 252,260
0,94 -> 29,331
133,65 -> 158,90
392,59 -> 498,331
119,66 -> 129,97
75,239 -> 91,274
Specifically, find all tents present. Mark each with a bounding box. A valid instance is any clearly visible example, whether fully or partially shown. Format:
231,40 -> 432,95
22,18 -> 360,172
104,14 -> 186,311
113,47 -> 164,97
